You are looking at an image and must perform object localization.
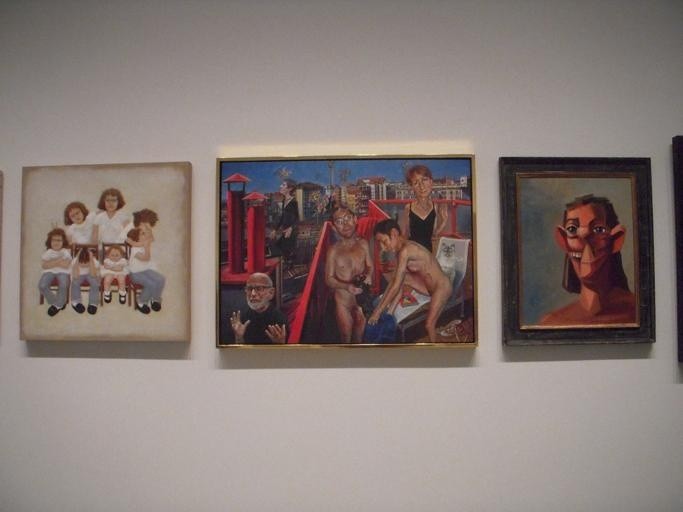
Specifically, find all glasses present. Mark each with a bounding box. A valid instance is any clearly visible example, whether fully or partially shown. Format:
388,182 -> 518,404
242,286 -> 272,293
333,213 -> 354,225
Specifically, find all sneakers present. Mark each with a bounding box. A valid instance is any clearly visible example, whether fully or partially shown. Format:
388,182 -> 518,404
48,304 -> 65,315
72,303 -> 85,313
87,304 -> 97,314
136,301 -> 150,313
119,293 -> 126,304
103,291 -> 111,303
151,300 -> 161,311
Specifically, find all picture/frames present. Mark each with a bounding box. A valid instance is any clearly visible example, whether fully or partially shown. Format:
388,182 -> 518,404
213,152 -> 480,351
497,153 -> 655,348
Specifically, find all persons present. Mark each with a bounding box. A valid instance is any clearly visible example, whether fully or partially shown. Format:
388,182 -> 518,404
324,165 -> 453,345
229,272 -> 290,344
36,187 -> 165,317
269,178 -> 301,266
